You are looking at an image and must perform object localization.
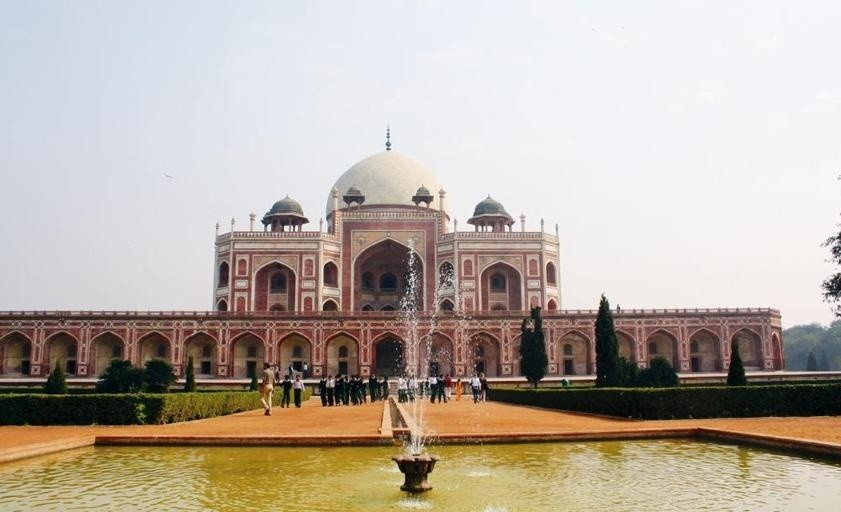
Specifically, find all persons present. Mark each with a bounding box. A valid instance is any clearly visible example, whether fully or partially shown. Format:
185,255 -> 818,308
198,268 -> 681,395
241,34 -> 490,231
275,364 -> 280,382
320,371 -> 490,406
260,362 -> 274,416
293,375 -> 305,408
303,362 -> 311,378
288,363 -> 295,380
282,374 -> 292,409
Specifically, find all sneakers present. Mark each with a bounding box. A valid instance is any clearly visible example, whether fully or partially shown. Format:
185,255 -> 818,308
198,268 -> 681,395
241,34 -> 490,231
281,403 -> 301,408
263,408 -> 272,416
